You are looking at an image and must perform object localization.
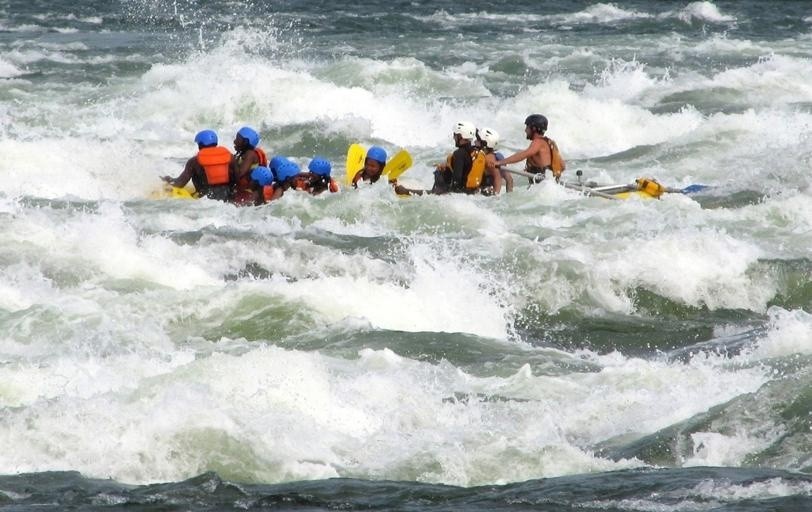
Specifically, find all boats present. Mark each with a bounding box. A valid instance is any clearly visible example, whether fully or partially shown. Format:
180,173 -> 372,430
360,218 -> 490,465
153,176 -> 665,207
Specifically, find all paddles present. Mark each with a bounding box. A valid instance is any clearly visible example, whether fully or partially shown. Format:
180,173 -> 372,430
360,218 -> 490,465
346,144 -> 366,187
381,151 -> 413,182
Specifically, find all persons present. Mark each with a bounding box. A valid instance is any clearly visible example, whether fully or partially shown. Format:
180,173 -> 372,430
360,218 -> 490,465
163,127 -> 339,207
487,114 -> 566,186
434,121 -> 514,195
351,146 -> 411,198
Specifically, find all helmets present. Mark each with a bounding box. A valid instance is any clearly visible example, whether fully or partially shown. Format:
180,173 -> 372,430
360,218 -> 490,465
249,154 -> 331,187
237,127 -> 259,147
452,120 -> 500,149
525,114 -> 548,130
195,130 -> 218,145
367,145 -> 386,164
